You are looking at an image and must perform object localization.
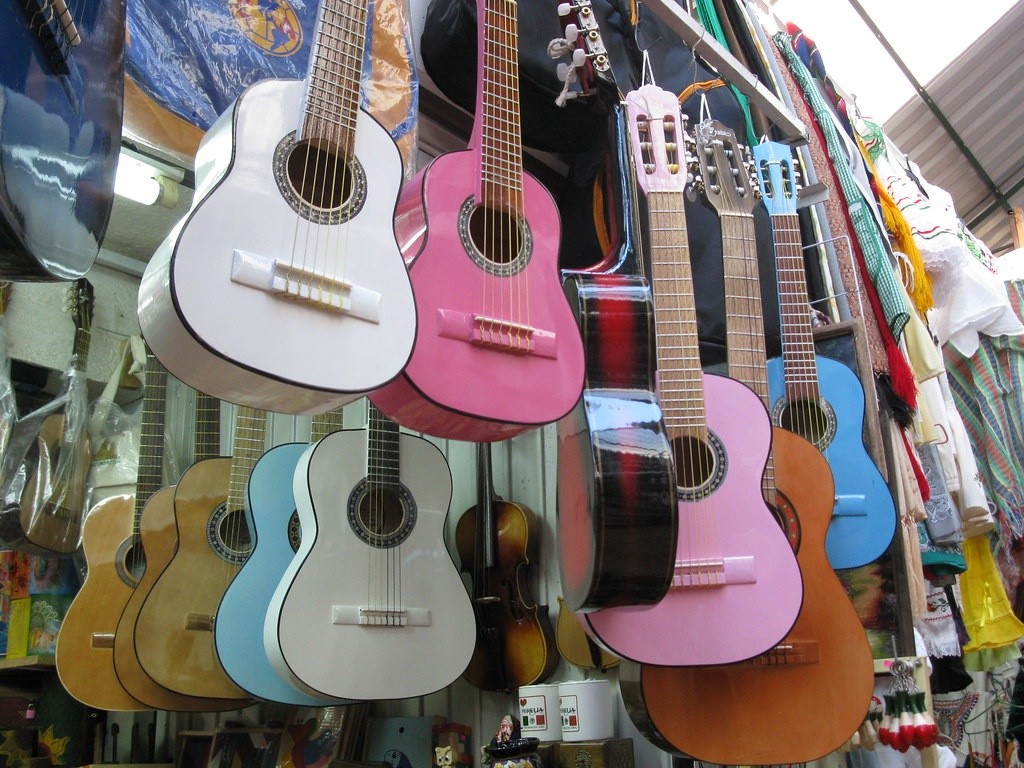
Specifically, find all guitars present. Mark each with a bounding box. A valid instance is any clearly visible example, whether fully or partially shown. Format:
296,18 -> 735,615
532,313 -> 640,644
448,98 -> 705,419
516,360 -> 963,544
365,0 -> 586,443
0,59 -> 902,768
136,0 -> 420,418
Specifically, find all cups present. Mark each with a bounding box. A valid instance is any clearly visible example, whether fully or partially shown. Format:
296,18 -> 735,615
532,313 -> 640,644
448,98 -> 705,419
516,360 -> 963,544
557,680 -> 616,743
515,683 -> 562,745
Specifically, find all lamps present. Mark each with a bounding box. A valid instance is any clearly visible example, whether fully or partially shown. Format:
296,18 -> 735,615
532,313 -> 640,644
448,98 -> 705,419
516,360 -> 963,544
114,140 -> 197,209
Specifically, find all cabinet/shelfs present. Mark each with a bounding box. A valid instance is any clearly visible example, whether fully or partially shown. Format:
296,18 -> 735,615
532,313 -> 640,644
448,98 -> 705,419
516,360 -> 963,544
0,387 -> 106,768
